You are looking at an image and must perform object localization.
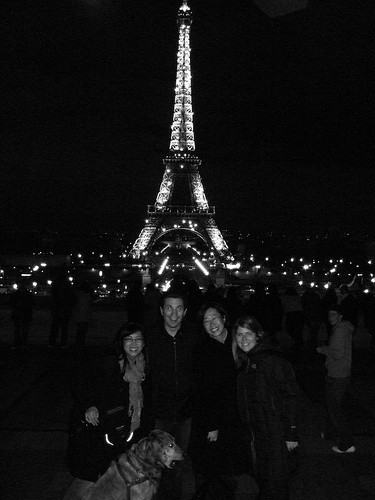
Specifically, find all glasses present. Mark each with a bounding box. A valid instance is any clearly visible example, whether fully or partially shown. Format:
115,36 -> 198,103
123,338 -> 144,343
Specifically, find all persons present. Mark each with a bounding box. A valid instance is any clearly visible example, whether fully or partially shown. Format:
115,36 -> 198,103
231,315 -> 313,500
1,258 -> 375,357
146,293 -> 207,500
314,307 -> 358,454
186,300 -> 249,500
84,319 -> 154,467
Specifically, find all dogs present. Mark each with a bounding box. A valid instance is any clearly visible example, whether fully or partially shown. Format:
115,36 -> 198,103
86,430 -> 185,500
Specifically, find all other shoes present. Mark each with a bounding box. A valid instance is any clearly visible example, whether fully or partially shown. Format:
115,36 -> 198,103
333,446 -> 355,453
